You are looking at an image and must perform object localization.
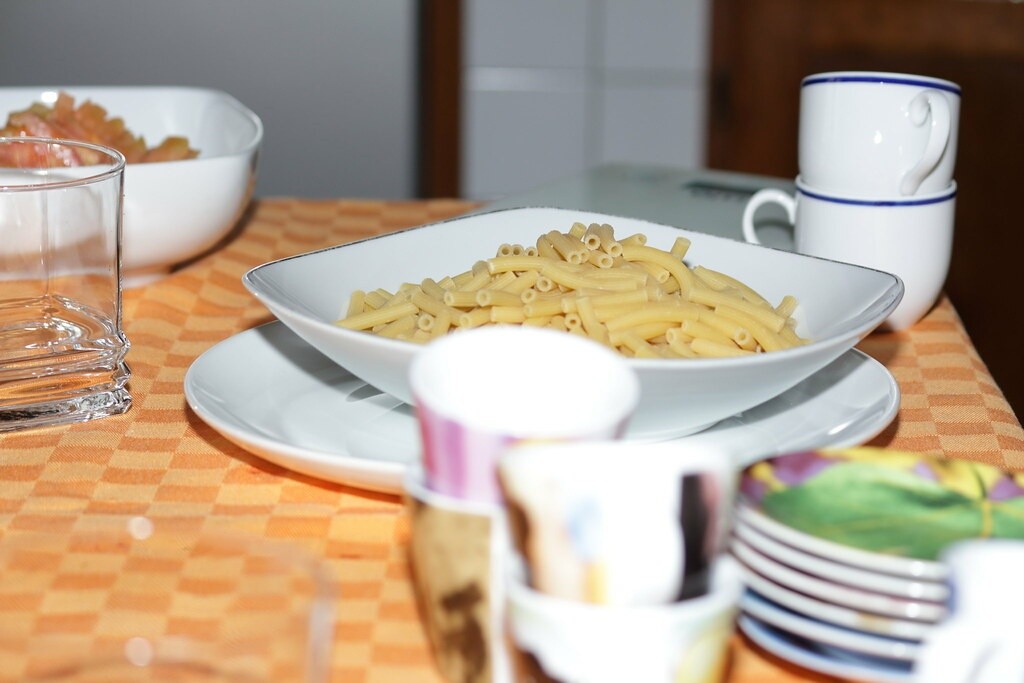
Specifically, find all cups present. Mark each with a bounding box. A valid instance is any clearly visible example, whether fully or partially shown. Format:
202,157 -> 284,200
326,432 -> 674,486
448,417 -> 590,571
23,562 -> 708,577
0,137 -> 132,433
408,325 -> 641,500
742,172 -> 958,330
397,472 -> 540,682
485,548 -> 746,683
798,72 -> 962,201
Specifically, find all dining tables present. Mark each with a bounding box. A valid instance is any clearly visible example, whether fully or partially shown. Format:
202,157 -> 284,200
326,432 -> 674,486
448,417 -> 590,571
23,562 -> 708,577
0,201 -> 1024,683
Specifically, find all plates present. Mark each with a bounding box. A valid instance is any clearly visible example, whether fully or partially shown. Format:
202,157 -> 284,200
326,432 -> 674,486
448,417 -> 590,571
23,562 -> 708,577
182,316 -> 901,496
730,447 -> 1023,682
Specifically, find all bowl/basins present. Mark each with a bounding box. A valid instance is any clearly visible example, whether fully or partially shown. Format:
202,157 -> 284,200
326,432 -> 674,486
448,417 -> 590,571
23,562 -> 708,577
0,85 -> 265,290
242,206 -> 903,442
